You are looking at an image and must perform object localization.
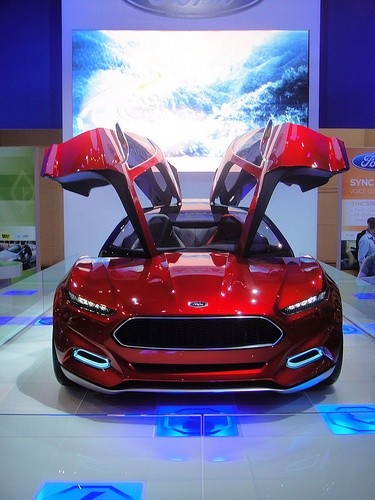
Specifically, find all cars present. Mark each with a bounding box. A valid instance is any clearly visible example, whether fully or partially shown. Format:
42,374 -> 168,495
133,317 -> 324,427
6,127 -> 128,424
0,245 -> 35,267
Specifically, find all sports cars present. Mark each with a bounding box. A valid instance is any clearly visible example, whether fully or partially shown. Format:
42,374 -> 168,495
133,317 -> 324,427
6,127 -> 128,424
39,118 -> 344,398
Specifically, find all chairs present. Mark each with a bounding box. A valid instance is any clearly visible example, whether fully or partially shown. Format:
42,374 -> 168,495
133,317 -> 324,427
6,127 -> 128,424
206,214 -> 262,245
130,214 -> 186,249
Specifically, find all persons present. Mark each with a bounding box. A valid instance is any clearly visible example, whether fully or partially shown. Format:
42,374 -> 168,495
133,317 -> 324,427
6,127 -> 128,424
356,217 -> 375,285
21,241 -> 32,268
